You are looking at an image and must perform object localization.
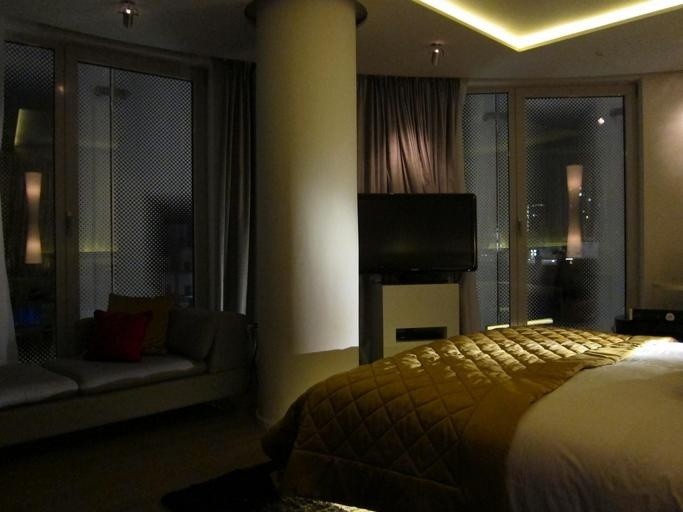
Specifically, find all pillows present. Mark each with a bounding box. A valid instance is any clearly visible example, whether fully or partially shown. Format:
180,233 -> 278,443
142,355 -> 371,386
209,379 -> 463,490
81,293 -> 176,362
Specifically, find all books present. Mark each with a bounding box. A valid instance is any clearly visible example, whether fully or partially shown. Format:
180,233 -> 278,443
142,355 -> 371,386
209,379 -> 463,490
632,307 -> 683,322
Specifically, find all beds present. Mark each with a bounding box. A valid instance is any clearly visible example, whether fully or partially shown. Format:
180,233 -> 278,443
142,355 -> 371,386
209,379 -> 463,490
271,325 -> 682,511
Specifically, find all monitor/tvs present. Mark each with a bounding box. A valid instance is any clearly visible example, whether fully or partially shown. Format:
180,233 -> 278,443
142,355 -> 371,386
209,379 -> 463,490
356,193 -> 478,284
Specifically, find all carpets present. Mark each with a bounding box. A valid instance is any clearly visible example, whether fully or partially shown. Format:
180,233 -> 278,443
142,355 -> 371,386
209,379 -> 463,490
158,460 -> 279,511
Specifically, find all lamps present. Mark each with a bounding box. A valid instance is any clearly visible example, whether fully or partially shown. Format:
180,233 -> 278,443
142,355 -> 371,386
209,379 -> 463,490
427,42 -> 444,69
117,1 -> 139,29
564,163 -> 584,259
21,169 -> 43,265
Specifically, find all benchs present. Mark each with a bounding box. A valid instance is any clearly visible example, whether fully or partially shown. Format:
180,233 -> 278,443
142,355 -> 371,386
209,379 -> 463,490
1,308 -> 248,463
